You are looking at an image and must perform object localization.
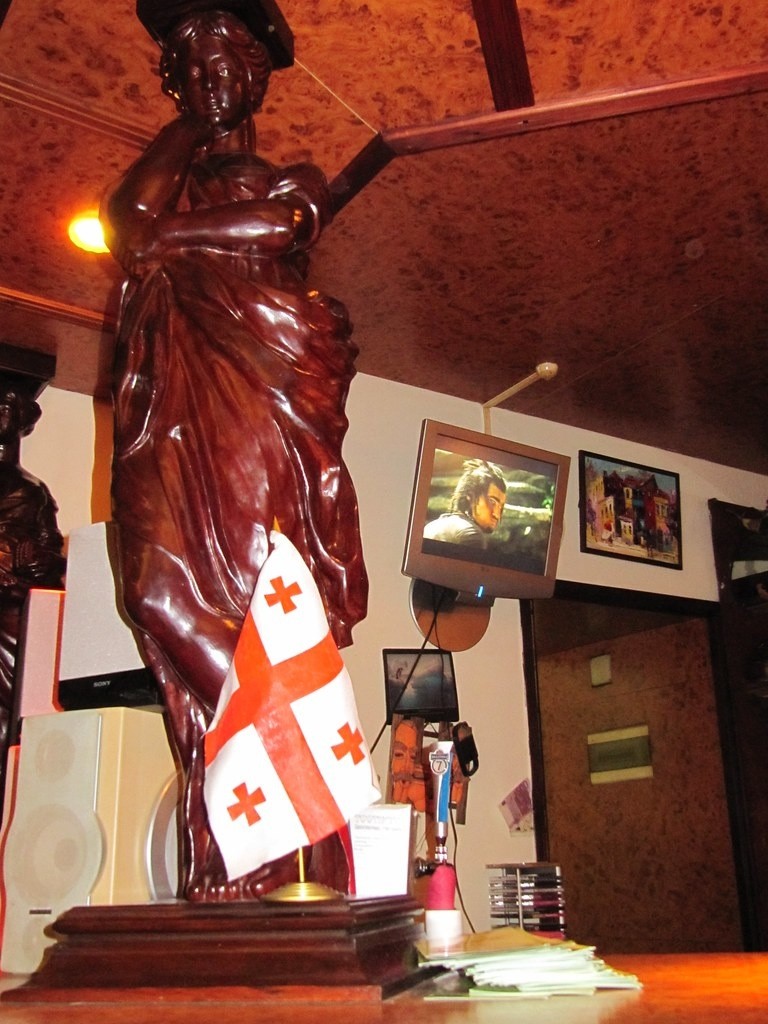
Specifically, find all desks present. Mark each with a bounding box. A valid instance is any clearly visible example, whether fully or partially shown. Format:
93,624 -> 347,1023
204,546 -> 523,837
0,951 -> 768,1024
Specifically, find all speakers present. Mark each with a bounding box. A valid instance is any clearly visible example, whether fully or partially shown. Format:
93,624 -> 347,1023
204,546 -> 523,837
0,706 -> 182,973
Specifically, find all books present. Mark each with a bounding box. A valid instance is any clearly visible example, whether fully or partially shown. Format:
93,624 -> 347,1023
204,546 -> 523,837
413,923 -> 644,1001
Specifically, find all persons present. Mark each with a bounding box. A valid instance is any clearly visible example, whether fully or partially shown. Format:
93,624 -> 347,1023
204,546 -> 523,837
98,9 -> 369,903
423,459 -> 507,549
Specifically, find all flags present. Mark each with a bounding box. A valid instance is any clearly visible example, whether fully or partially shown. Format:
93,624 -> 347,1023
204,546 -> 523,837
203,530 -> 383,881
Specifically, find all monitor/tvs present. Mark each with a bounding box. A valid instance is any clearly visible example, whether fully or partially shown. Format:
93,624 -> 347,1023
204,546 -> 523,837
402,418 -> 571,607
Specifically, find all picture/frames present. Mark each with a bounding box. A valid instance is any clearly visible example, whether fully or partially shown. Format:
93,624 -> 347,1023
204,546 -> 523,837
382,648 -> 460,727
580,449 -> 684,571
